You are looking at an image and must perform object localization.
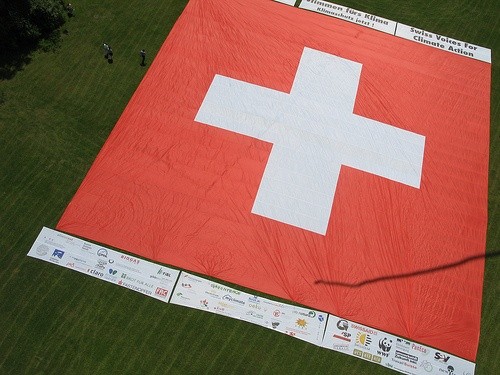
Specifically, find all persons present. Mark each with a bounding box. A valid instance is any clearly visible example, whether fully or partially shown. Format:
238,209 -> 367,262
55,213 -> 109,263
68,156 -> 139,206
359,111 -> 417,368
104,41 -> 110,53
140,49 -> 146,66
107,46 -> 114,64
